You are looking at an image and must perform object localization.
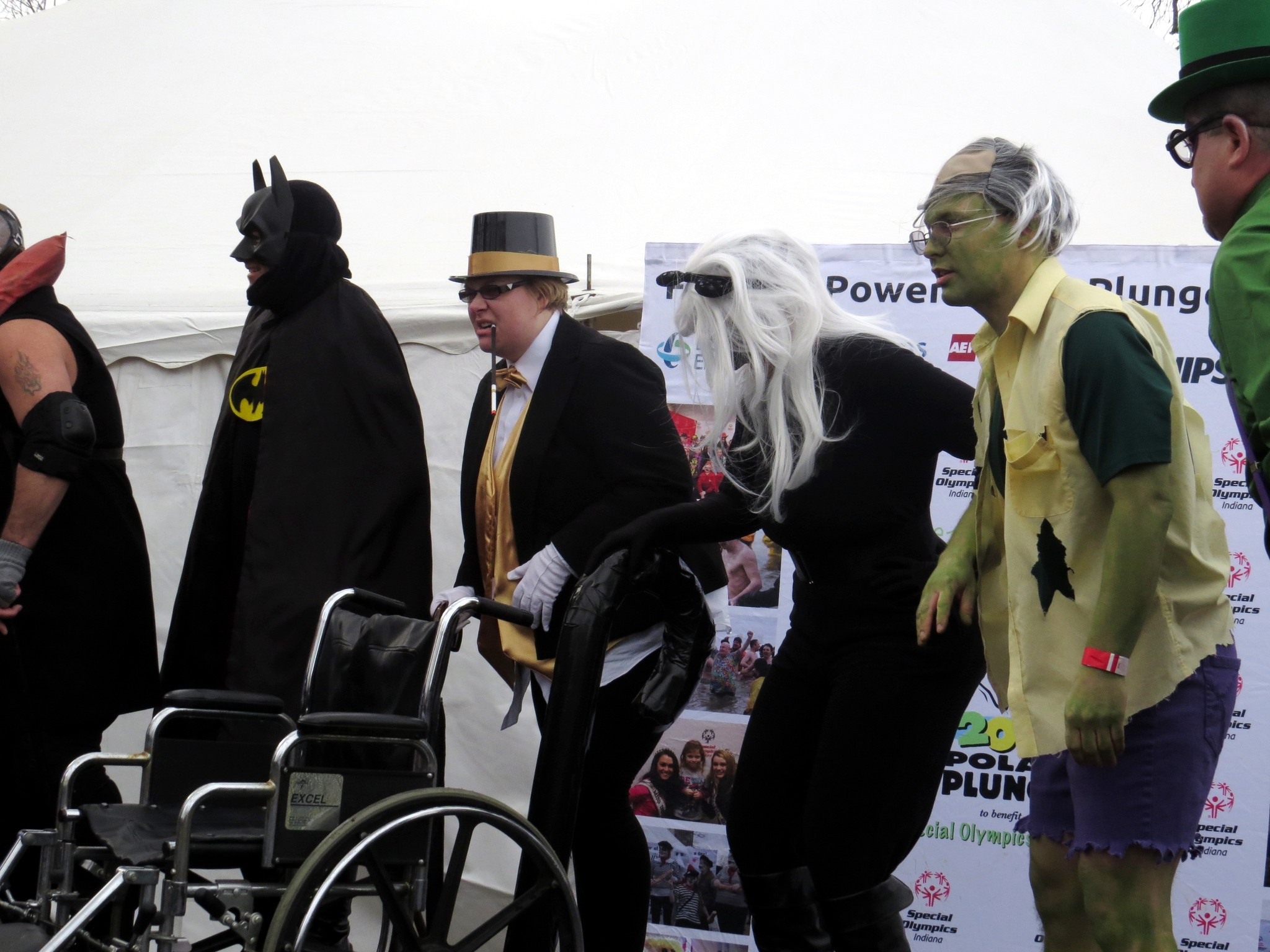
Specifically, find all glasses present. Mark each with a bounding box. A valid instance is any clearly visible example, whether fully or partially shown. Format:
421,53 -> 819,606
458,279 -> 528,303
908,215 -> 1002,255
1166,112 -> 1270,169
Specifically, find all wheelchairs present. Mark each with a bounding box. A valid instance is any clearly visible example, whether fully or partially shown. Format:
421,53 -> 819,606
0,588 -> 584,952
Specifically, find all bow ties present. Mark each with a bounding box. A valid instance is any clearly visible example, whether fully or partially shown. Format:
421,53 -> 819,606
491,367 -> 527,391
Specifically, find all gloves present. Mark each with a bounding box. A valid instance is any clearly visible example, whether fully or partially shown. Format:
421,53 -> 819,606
430,586 -> 476,633
507,540 -> 578,633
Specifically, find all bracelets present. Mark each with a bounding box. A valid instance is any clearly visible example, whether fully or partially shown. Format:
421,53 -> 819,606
1075,647 -> 1132,676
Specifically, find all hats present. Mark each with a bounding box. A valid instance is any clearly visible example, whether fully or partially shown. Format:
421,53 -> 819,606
721,640 -> 729,643
722,432 -> 728,438
683,869 -> 699,876
733,637 -> 742,643
679,433 -> 689,437
1147,0 -> 1269,124
448,211 -> 579,284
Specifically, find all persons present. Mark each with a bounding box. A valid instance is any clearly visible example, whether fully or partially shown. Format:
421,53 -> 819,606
443,202 -> 717,952
0,202 -> 162,945
605,400 -> 811,952
1150,2 -> 1270,552
581,225 -> 1001,952
907,138 -> 1241,952
153,154 -> 452,952
